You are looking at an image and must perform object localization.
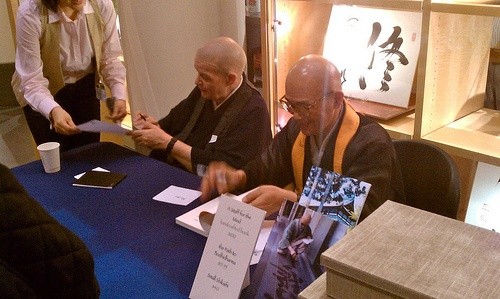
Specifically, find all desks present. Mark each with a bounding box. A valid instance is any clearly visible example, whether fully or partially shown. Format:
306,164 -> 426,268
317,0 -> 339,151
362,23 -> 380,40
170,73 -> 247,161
8,142 -> 256,298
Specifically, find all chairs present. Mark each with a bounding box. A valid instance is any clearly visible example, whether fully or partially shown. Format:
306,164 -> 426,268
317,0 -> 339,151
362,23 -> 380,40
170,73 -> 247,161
392,139 -> 461,220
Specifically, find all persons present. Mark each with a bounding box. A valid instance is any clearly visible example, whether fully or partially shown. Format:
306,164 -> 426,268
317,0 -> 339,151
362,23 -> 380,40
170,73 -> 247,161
130,37 -> 272,175
214,54 -> 406,224
276,214 -> 313,269
11,0 -> 127,151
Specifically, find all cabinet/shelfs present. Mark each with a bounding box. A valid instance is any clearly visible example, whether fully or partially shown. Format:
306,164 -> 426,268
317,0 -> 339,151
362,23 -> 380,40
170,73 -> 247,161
260,0 -> 500,223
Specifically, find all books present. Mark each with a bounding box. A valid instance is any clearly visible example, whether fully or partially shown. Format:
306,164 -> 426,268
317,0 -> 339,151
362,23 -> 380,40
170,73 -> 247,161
176,191 -> 255,238
73,170 -> 128,189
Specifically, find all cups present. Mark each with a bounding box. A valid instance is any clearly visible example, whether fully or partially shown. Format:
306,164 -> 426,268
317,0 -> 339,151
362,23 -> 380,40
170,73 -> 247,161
37,142 -> 60,173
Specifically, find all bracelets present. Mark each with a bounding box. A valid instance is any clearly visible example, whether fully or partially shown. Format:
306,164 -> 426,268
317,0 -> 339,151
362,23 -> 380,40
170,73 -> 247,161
165,137 -> 178,154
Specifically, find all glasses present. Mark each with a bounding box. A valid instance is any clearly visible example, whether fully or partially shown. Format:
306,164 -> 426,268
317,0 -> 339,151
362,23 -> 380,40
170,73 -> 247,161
280,88 -> 336,120
300,221 -> 309,227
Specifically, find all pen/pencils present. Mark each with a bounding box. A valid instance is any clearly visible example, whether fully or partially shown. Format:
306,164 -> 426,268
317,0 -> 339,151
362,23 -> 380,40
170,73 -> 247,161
140,114 -> 146,120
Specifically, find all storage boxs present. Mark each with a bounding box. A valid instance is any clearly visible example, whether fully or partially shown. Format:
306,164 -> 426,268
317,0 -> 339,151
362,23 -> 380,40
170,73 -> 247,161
319,199 -> 500,299
